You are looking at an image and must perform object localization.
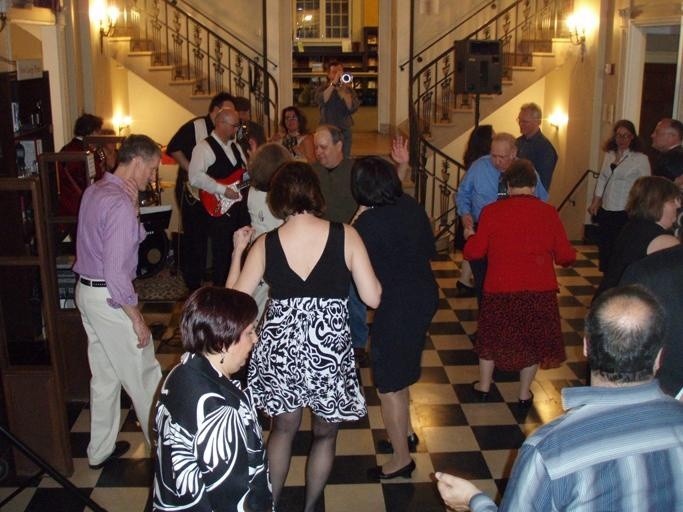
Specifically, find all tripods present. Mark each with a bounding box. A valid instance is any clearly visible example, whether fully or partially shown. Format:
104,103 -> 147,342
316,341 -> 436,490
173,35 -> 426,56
434,97 -> 481,244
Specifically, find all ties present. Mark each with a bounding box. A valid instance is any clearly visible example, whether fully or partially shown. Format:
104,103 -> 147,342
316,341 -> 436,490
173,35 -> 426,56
497,171 -> 509,200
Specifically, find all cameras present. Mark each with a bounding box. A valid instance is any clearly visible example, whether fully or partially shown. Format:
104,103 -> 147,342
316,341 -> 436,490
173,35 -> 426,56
342,73 -> 354,84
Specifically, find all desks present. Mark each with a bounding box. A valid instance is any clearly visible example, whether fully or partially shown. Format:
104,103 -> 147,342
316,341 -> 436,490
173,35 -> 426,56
292,72 -> 377,107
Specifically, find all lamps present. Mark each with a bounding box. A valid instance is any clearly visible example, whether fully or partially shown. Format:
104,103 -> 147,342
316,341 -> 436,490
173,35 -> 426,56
109,114 -> 132,136
565,6 -> 594,56
90,2 -> 121,35
546,113 -> 564,133
6,0 -> 58,24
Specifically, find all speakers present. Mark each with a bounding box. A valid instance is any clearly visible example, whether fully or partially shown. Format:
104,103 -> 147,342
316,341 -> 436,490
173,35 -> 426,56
454,40 -> 502,95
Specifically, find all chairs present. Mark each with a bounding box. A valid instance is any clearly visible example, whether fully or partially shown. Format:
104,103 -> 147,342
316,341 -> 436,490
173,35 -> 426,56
133,231 -> 188,354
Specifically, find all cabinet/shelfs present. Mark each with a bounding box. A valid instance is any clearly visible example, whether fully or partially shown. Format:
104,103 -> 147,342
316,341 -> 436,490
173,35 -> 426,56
0,71 -> 90,478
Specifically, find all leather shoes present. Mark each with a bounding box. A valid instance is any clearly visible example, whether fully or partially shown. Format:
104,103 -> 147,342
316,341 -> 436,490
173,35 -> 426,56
377,431 -> 420,454
369,454 -> 415,483
516,389 -> 534,409
466,377 -> 489,400
86,438 -> 131,472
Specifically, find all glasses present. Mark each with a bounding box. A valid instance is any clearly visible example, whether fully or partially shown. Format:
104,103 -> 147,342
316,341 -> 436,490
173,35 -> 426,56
616,131 -> 633,139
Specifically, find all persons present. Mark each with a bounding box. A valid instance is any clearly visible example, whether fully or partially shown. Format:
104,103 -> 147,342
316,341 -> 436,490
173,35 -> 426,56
435,281 -> 682,512
452,102 -> 576,423
588,119 -> 683,398
50,59 -> 440,511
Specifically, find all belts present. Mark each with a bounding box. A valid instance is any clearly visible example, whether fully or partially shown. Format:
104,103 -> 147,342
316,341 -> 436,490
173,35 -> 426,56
76,277 -> 108,288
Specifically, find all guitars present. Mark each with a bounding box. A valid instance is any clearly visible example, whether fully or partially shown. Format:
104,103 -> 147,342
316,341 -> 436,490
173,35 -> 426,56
200,169 -> 253,217
